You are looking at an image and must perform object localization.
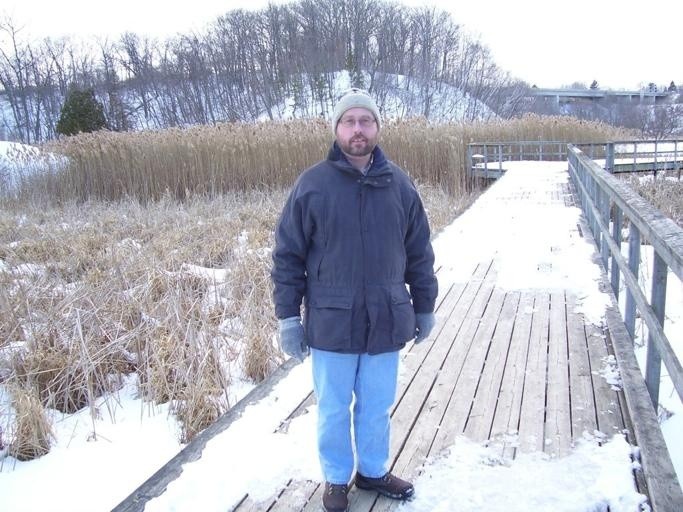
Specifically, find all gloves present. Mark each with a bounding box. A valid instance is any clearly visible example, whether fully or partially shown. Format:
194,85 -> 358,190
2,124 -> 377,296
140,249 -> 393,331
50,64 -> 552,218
279,316 -> 309,362
412,312 -> 435,344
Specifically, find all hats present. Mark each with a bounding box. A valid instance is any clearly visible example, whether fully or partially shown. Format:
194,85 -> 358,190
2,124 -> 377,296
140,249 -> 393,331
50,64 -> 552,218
331,87 -> 381,136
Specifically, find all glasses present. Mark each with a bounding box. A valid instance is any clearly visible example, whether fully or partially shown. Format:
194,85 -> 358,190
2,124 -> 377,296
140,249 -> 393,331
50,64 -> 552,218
339,115 -> 376,127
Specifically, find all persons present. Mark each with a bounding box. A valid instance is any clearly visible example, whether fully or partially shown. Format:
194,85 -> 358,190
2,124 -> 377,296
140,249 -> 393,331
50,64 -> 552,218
268,91 -> 440,512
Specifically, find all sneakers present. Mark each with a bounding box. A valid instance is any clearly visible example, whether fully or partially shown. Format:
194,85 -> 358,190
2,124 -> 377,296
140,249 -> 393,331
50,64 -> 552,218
321,481 -> 348,512
355,471 -> 415,499
